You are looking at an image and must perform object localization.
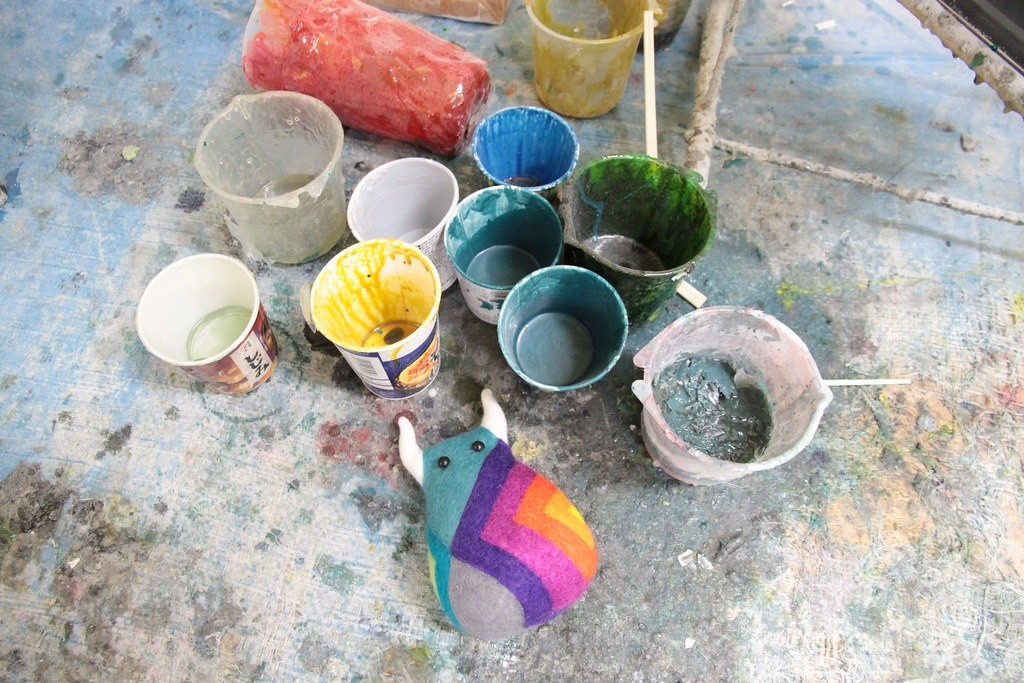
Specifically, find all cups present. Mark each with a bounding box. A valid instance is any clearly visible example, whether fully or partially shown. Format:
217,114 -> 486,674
525,0 -> 659,118
241,1 -> 493,157
346,157 -> 459,290
135,254 -> 279,398
471,107 -> 580,207
638,0 -> 692,55
498,265 -> 628,392
309,240 -> 443,399
444,186 -> 562,325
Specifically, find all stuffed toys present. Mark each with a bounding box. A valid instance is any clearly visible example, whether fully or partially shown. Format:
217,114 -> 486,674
396,389 -> 597,641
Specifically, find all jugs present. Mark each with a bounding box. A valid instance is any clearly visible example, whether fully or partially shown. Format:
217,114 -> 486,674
639,306 -> 835,487
562,154 -> 716,333
194,91 -> 344,264
